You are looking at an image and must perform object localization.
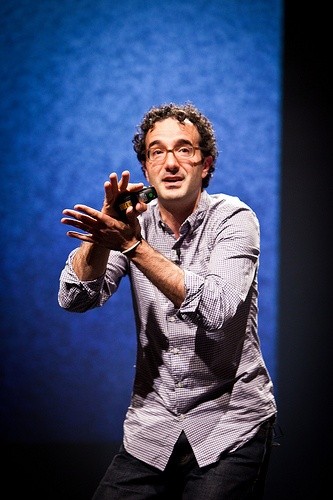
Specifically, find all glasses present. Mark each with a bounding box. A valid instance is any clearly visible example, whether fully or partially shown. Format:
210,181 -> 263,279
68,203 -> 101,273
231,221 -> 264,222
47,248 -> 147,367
144,144 -> 204,161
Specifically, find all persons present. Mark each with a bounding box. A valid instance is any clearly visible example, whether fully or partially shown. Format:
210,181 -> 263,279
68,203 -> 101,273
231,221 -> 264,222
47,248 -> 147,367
56,101 -> 278,500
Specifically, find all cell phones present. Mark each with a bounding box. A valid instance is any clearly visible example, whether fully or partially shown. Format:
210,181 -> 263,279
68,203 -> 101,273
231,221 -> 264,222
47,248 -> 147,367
115,187 -> 158,215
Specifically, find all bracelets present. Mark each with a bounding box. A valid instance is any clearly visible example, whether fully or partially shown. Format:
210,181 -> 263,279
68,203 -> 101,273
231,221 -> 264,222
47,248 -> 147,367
120,234 -> 143,254
